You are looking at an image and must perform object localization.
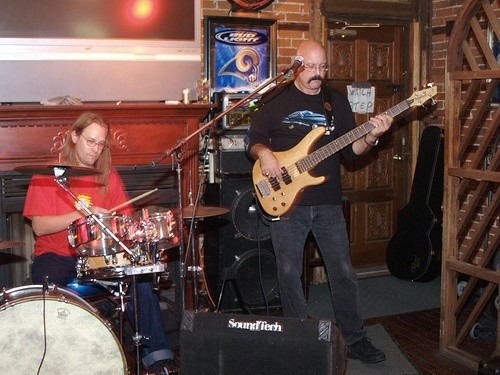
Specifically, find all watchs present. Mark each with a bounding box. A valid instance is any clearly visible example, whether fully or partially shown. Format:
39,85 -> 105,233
364,135 -> 378,146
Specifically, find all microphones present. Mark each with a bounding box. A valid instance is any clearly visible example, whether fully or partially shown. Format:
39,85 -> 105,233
284,55 -> 305,81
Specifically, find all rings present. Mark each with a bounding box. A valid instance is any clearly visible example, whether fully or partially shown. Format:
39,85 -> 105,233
266,174 -> 269,176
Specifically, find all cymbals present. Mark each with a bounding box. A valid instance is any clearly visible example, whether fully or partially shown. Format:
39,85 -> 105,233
12,162 -> 104,176
170,205 -> 229,219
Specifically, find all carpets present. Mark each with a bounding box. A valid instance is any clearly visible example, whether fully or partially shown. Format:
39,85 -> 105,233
343,324 -> 422,375
306,274 -> 441,323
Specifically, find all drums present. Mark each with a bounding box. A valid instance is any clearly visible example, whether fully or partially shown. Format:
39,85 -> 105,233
145,210 -> 179,251
0,281 -> 128,375
67,211 -> 137,257
73,247 -> 151,280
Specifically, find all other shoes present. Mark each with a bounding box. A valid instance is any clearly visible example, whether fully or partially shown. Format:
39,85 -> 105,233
147,359 -> 181,375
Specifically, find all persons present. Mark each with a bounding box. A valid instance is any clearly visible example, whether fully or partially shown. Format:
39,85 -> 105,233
23,113 -> 175,375
245,40 -> 386,363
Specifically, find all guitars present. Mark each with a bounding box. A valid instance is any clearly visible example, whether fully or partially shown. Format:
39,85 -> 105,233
252,80 -> 438,217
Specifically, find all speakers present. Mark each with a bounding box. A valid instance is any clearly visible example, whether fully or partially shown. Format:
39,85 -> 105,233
202,176 -> 306,311
180,310 -> 350,375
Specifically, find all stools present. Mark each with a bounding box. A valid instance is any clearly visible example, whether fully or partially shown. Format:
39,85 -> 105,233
66,277 -> 109,302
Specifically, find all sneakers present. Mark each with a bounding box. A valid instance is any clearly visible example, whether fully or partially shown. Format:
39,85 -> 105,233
347,336 -> 386,363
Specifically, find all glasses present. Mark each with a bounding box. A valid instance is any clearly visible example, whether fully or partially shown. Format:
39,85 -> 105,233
80,133 -> 108,149
303,65 -> 329,71
